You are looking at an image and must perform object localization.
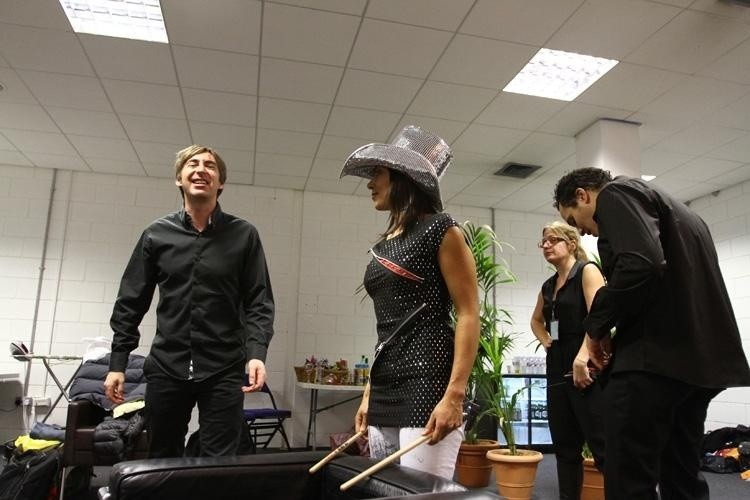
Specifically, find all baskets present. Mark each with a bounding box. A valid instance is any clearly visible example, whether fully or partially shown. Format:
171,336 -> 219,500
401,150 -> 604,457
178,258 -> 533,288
299,367 -> 347,386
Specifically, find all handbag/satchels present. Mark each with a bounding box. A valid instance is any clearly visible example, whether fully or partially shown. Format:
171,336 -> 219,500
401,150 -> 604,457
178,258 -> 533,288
0,433 -> 64,500
703,424 -> 750,474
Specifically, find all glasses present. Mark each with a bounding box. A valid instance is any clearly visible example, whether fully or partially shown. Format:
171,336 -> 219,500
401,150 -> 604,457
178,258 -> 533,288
538,237 -> 564,248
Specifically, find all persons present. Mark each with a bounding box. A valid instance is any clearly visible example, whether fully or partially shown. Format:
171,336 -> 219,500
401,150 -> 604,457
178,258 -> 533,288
337,123 -> 481,482
553,166 -> 749,500
102,144 -> 275,458
529,222 -> 609,500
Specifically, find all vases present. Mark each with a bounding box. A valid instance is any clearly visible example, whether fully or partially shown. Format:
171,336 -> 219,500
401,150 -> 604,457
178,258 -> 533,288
580,441 -> 605,500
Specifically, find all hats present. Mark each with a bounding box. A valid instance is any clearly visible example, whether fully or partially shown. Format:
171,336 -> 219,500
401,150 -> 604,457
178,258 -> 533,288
339,124 -> 454,212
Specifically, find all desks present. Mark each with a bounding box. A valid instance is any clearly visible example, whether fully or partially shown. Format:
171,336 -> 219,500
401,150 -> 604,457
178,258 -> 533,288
296,381 -> 366,451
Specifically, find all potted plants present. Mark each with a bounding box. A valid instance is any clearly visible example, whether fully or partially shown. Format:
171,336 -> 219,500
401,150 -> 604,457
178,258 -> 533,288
449,222 -> 544,500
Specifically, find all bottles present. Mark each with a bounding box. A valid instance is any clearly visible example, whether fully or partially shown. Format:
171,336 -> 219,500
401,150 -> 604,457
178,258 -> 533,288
511,356 -> 547,375
355,355 -> 369,386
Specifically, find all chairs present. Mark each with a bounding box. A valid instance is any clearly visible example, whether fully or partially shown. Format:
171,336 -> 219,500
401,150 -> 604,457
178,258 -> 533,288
66,400 -> 149,464
111,450 -> 468,500
243,375 -> 292,453
